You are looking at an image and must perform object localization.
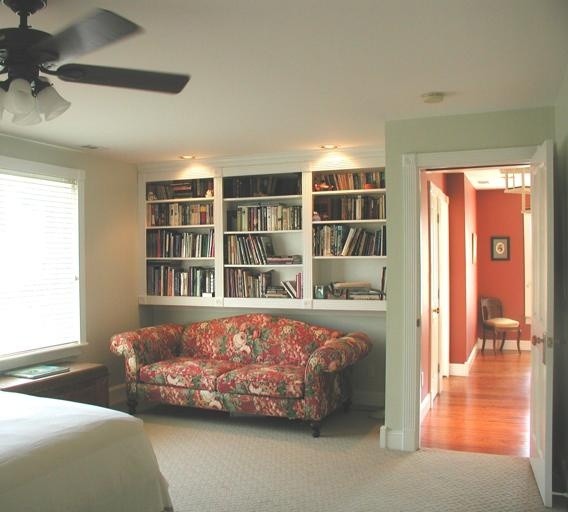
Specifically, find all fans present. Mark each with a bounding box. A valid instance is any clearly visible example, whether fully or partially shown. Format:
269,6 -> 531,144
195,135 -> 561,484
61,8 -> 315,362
0,0 -> 191,95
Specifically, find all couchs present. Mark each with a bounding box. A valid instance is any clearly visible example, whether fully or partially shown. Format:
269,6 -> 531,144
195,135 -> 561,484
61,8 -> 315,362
109,312 -> 372,443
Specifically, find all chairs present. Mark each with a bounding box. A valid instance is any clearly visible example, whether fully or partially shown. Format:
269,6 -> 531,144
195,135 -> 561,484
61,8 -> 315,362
480,296 -> 523,356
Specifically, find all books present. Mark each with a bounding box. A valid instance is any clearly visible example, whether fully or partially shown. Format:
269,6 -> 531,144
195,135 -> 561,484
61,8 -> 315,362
146,178 -> 215,296
4,364 -> 70,379
223,178 -> 302,298
313,280 -> 385,300
312,170 -> 386,256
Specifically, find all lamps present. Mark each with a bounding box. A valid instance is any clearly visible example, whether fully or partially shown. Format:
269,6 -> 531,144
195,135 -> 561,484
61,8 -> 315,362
0,63 -> 72,127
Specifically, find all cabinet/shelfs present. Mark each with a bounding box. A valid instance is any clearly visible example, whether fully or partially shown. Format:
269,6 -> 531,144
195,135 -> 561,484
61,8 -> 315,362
138,163 -> 386,309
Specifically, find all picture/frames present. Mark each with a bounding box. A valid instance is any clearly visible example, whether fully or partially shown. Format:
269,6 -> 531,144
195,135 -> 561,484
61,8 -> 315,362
491,236 -> 510,261
